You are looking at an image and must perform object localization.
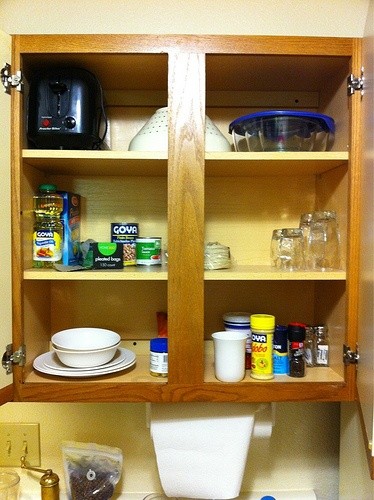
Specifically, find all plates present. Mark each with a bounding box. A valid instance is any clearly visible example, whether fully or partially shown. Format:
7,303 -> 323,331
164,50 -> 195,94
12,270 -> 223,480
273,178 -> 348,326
32,346 -> 137,377
202,245 -> 231,271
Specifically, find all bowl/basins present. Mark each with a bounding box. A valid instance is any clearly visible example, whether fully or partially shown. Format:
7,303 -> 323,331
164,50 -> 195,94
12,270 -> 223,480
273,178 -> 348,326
50,326 -> 121,368
229,108 -> 336,152
129,105 -> 231,153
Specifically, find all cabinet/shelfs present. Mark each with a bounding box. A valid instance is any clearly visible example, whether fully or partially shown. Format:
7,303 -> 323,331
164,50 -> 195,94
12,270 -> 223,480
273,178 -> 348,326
0,0 -> 374,481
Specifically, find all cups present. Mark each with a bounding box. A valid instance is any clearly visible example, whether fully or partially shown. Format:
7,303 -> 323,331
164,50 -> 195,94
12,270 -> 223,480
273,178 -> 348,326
271,226 -> 307,272
142,493 -> 178,500
211,331 -> 250,382
299,209 -> 342,274
0,468 -> 20,500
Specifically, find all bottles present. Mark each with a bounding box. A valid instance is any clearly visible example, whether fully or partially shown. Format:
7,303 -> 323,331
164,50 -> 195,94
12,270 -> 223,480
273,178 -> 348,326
304,325 -> 329,367
249,314 -> 274,380
287,321 -> 306,377
222,311 -> 253,369
41,468 -> 62,500
149,338 -> 168,377
31,183 -> 64,269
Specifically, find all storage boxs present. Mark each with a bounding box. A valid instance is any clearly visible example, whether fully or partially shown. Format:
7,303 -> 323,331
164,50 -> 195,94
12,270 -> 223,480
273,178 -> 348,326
57,191 -> 81,266
